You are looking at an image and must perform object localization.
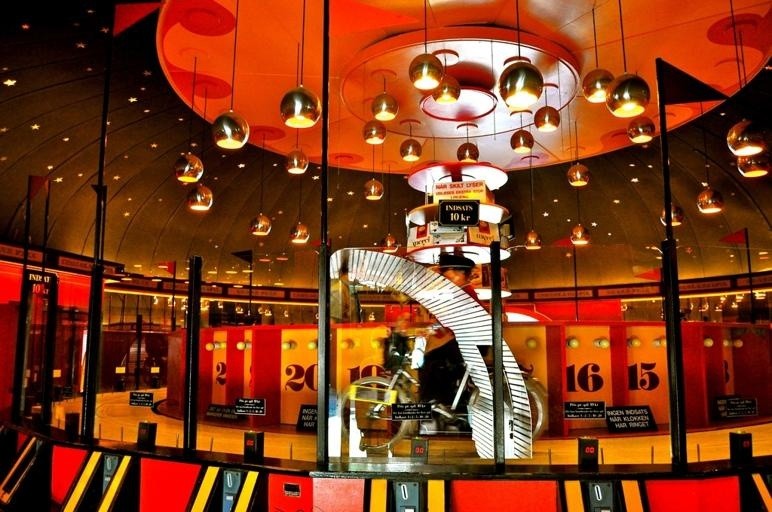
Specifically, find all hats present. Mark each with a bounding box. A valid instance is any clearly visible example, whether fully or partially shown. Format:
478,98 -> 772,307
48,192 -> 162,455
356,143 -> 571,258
429,251 -> 475,269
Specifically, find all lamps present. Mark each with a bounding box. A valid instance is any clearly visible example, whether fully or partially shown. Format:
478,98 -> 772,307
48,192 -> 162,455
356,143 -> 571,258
408,1 -> 443,90
173,56 -> 204,183
284,44 -> 308,175
582,9 -> 617,103
737,31 -> 772,178
606,1 -> 651,118
570,151 -> 590,245
626,70 -> 655,144
371,78 -> 399,121
186,88 -> 212,211
433,1 -> 458,97
499,0 -> 544,107
400,123 -> 423,161
279,0 -> 321,128
510,116 -> 534,153
695,102 -> 725,214
524,163 -> 543,250
288,147 -> 310,243
660,157 -> 684,227
380,164 -> 398,253
566,121 -> 590,186
362,101 -> 386,145
456,128 -> 480,163
726,3 -> 766,157
363,101 -> 385,201
212,0 -> 250,150
250,133 -> 272,236
535,91 -> 560,132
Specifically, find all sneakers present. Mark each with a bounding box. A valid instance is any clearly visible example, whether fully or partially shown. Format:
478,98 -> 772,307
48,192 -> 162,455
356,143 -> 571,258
427,398 -> 457,421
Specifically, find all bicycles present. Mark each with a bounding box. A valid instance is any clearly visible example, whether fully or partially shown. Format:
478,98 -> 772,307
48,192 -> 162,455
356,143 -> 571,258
338,324 -> 548,455
